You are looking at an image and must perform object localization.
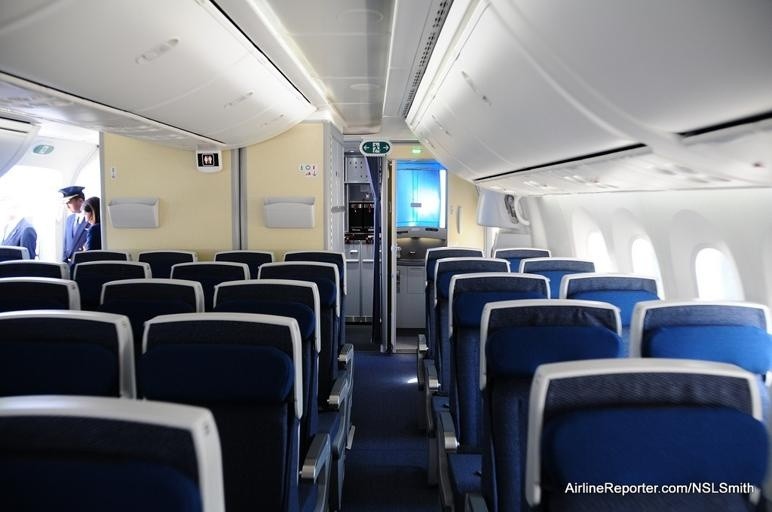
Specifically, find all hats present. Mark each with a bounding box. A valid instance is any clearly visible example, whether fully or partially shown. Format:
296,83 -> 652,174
59,187 -> 85,205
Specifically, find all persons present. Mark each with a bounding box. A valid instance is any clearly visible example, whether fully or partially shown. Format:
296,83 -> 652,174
58,186 -> 90,263
80,198 -> 98,251
1,216 -> 37,259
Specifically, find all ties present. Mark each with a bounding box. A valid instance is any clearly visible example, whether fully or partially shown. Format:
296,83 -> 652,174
74,217 -> 80,234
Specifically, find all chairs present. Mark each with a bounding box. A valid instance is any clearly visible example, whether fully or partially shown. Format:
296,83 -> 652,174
70,249 -> 130,263
559,273 -> 659,340
138,250 -> 198,279
416,246 -> 484,391
465,298 -> 624,512
430,271 -> 551,512
0,275 -> 81,310
135,312 -> 333,512
627,298 -> 772,409
213,250 -> 274,280
211,278 -> 353,512
0,244 -> 30,261
256,260 -> 356,450
520,357 -> 768,512
168,260 -> 251,312
0,310 -> 134,400
73,260 -> 152,310
282,250 -> 348,348
421,256 -> 511,438
493,247 -> 551,271
98,277 -> 206,322
0,393 -> 226,512
518,256 -> 595,298
0,259 -> 70,281
